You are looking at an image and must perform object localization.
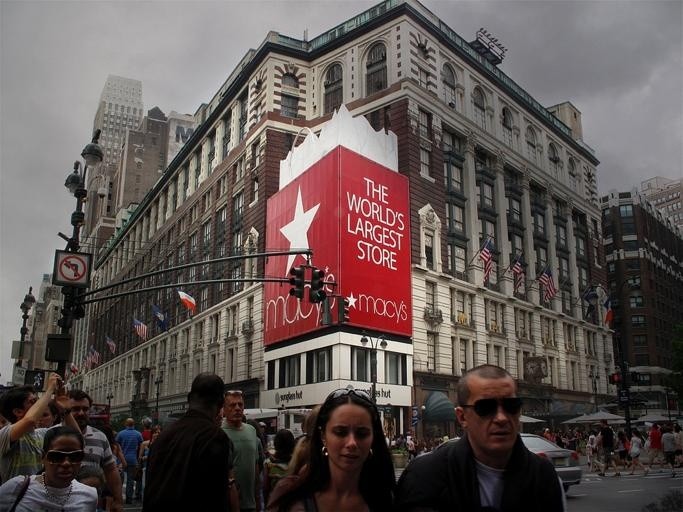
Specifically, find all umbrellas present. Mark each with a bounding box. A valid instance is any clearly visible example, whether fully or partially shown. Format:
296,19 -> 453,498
563,411 -> 676,427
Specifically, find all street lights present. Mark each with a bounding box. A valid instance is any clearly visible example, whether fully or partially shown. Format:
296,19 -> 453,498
587,369 -> 600,413
49,125 -> 104,390
154,373 -> 163,420
360,327 -> 390,406
586,274 -> 642,439
106,392 -> 113,425
647,386 -> 681,423
15,286 -> 35,366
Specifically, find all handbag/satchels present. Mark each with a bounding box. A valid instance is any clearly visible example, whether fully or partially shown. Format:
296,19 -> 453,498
630,447 -> 639,458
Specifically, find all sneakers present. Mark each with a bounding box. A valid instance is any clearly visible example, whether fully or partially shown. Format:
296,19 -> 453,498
643,468 -> 648,476
671,471 -> 676,478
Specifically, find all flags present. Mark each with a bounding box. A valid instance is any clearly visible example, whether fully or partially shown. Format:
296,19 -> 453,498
108,337 -> 118,356
605,299 -> 615,326
510,256 -> 529,292
535,267 -> 558,304
585,288 -> 600,320
151,306 -> 169,333
176,288 -> 198,319
92,349 -> 100,364
83,356 -> 92,370
134,320 -> 149,342
476,239 -> 492,272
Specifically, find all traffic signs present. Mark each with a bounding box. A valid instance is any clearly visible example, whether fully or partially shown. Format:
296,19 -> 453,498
11,365 -> 27,387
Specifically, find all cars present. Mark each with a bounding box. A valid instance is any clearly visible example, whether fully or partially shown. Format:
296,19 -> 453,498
415,433 -> 580,495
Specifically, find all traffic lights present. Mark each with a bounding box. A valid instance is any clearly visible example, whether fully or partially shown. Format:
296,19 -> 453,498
337,297 -> 349,323
311,267 -> 326,304
288,266 -> 304,299
607,371 -> 621,384
24,370 -> 45,393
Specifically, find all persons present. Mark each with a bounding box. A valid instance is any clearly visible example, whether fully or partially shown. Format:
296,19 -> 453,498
396,364 -> 564,511
217,388 -> 323,511
267,387 -> 396,511
540,419 -> 683,478
536,452 -> 569,511
392,430 -> 447,462
0,369 -> 161,512
142,372 -> 234,512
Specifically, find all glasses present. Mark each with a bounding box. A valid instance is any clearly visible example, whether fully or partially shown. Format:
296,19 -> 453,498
30,399 -> 38,405
45,450 -> 86,466
225,390 -> 243,397
327,388 -> 370,402
461,396 -> 522,417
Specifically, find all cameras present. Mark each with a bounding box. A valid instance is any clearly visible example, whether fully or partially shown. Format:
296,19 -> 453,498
56,378 -> 65,389
